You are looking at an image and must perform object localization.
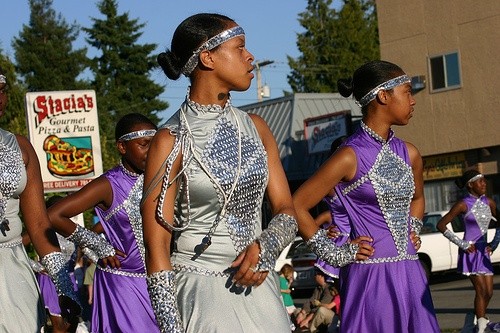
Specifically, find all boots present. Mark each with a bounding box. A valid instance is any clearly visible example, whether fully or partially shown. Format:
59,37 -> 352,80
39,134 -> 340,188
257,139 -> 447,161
473,314 -> 490,333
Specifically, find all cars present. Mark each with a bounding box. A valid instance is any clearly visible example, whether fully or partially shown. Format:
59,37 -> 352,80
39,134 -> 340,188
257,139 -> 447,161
416,209 -> 500,280
274,237 -> 334,292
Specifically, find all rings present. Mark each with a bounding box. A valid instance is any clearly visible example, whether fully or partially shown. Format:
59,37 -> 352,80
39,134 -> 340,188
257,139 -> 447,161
249,267 -> 256,274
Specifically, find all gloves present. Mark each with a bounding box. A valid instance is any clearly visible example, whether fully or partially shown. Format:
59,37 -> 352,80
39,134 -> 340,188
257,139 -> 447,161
314,299 -> 320,306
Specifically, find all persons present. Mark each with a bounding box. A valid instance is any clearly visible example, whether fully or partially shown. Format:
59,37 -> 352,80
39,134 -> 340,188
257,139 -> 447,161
139,12 -> 299,333
0,72 -> 83,333
436,170 -> 500,333
19,133 -> 357,333
292,60 -> 443,333
46,113 -> 160,333
